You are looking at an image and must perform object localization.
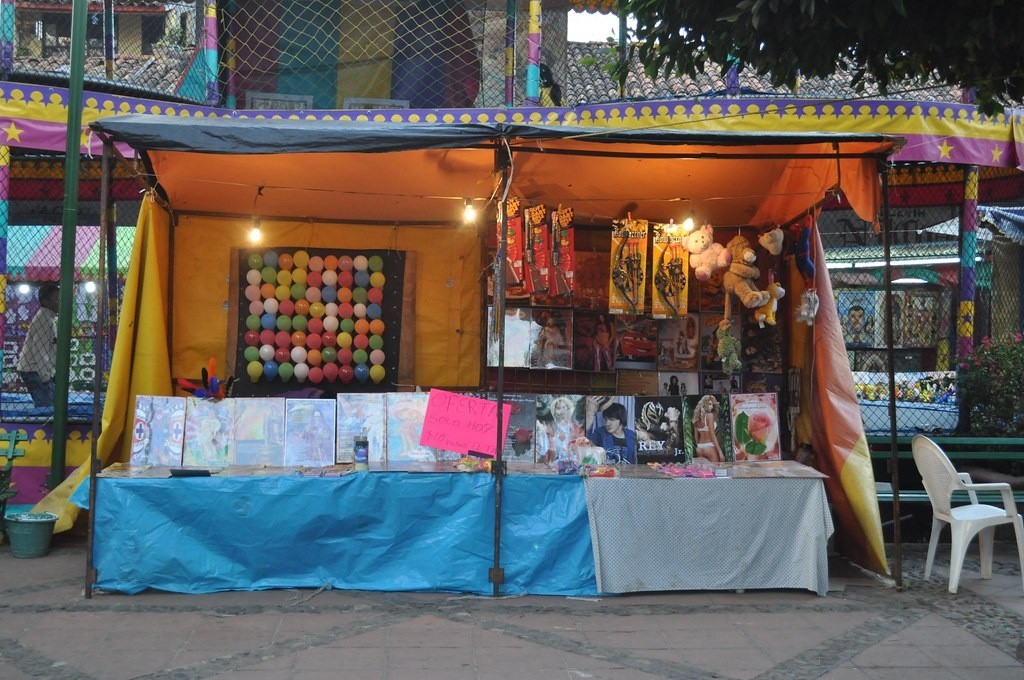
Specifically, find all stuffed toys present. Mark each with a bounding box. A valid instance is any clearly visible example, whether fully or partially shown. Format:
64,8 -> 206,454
686,223 -> 820,327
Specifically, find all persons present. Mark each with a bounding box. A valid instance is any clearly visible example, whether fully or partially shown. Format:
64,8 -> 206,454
18,281 -> 60,409
300,407 -> 334,465
844,304 -> 872,343
748,377 -> 782,394
864,314 -> 873,332
748,313 -> 783,326
594,310 -> 615,371
538,316 -> 565,366
749,361 -> 782,373
838,316 -> 849,335
742,342 -> 781,361
691,396 -> 726,462
586,395 -> 636,465
546,395 -> 583,461
636,401 -> 680,458
744,325 -> 780,341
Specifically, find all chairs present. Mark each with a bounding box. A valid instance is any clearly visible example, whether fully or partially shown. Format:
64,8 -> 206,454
911,434 -> 1024,593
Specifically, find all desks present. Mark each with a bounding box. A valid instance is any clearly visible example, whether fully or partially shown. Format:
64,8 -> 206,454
66,463 -> 836,597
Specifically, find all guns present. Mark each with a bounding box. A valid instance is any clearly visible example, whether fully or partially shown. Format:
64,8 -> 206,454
552,210 -> 571,298
501,250 -> 521,289
526,208 -> 547,296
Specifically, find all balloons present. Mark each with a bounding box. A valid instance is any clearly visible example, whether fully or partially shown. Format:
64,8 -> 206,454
244,250 -> 386,382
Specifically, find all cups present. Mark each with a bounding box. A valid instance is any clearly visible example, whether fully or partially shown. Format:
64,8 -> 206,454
354,440 -> 368,470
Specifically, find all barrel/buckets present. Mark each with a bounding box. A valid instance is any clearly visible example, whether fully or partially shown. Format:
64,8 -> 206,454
4,511 -> 59,559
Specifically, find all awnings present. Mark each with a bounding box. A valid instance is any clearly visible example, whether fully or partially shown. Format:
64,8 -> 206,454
90,114 -> 906,232
